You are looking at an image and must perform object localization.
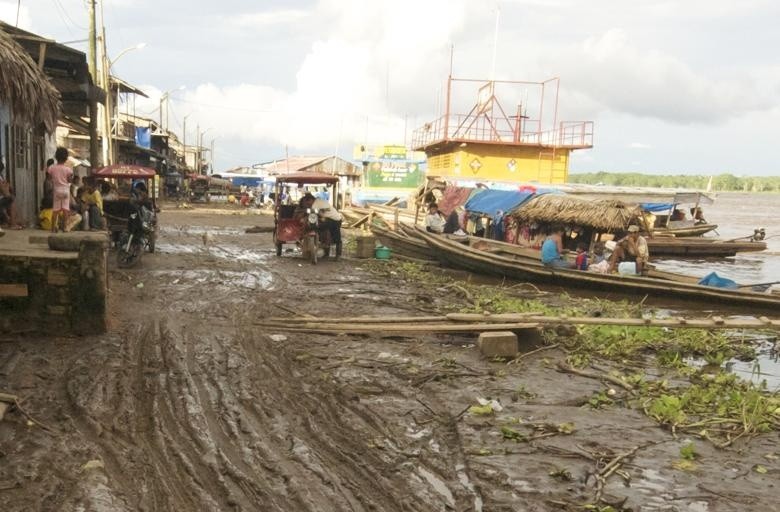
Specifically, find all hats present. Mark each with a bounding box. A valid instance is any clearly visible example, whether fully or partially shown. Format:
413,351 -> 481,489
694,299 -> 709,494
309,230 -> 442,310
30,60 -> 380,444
627,224 -> 639,233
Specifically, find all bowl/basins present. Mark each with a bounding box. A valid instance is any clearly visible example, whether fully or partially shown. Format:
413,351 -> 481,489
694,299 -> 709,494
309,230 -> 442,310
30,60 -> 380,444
375,248 -> 392,259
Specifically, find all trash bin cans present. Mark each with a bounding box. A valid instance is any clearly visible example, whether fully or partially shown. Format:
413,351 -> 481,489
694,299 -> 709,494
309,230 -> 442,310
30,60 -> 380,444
354,236 -> 377,258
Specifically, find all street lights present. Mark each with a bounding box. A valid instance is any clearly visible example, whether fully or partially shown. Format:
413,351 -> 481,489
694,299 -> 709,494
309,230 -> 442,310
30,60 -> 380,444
158,84 -> 221,179
105,43 -> 149,165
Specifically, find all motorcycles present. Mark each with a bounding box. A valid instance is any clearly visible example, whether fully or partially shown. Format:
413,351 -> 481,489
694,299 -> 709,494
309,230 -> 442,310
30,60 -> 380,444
271,171 -> 341,264
115,197 -> 160,270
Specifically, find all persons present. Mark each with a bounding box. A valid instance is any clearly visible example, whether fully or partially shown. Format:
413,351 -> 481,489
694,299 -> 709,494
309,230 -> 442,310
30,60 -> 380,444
538,220 -> 575,270
226,191 -> 236,203
423,202 -> 467,236
606,224 -> 649,273
573,242 -> 587,271
0,156 -> 25,234
241,189 -> 267,207
41,147 -> 160,236
303,192 -> 344,260
